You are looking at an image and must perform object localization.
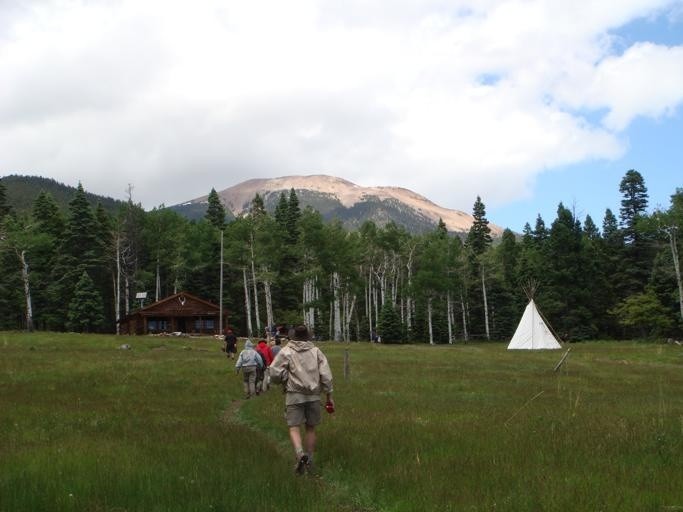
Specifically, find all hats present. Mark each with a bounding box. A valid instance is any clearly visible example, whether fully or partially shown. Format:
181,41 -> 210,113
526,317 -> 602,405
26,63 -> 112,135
288,325 -> 312,341
259,338 -> 265,342
245,339 -> 253,347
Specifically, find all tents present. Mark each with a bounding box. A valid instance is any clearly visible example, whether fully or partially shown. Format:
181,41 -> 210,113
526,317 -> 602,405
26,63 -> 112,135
507,299 -> 562,350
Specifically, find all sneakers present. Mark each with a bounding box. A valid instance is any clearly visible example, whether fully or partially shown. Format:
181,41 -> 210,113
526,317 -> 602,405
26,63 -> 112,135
245,385 -> 269,400
296,453 -> 312,474
227,355 -> 234,360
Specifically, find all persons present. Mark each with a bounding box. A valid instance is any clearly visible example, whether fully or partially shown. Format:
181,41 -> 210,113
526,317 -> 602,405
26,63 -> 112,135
224,329 -> 282,399
270,324 -> 336,474
